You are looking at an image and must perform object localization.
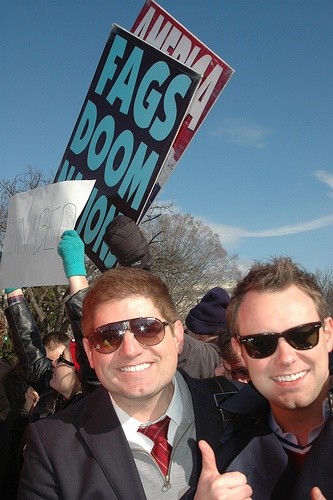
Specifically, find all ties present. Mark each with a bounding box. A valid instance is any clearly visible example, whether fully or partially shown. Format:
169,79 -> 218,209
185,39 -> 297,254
137,416 -> 174,477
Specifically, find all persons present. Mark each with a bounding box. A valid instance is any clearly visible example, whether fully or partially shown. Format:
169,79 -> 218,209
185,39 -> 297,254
180,257 -> 333,500
0,229 -> 96,477
105,215 -> 229,379
19,268 -> 251,500
0,364 -> 41,500
43,332 -> 71,363
214,320 -> 250,384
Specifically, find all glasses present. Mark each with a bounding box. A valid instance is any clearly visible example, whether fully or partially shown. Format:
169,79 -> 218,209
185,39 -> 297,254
57,354 -> 75,367
235,321 -> 324,359
85,316 -> 170,354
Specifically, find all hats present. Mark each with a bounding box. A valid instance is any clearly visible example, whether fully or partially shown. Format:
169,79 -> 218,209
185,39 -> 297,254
185,287 -> 233,336
69,341 -> 80,372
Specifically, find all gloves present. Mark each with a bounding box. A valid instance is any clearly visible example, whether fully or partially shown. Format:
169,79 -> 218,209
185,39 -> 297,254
102,215 -> 156,271
56,229 -> 87,278
